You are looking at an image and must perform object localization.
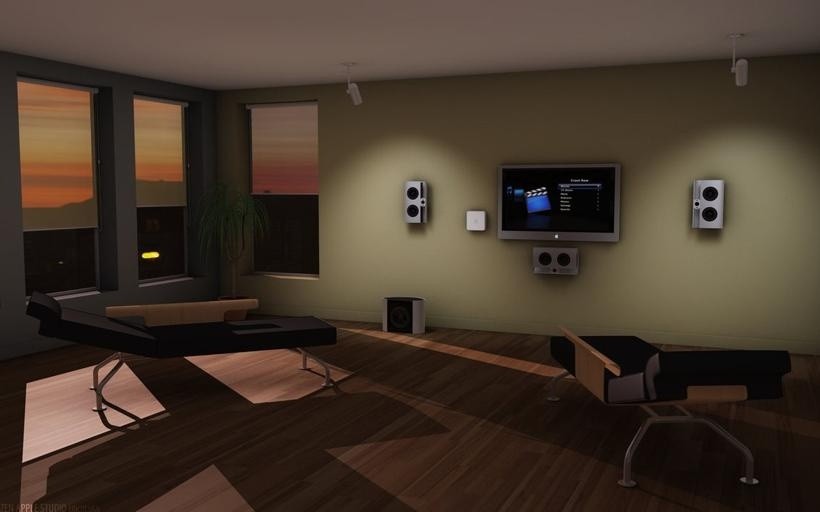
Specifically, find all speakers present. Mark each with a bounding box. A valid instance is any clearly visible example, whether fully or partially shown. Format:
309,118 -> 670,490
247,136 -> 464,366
532,247 -> 580,276
691,178 -> 724,230
383,296 -> 426,336
403,180 -> 428,224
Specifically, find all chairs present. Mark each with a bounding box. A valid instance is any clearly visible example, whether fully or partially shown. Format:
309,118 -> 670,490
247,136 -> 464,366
549,321 -> 793,487
30,289 -> 337,435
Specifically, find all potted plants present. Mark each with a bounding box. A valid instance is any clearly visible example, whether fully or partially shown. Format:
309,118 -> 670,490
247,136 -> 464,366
189,184 -> 270,321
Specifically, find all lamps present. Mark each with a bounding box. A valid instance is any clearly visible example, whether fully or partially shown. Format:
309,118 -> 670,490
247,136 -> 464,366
342,61 -> 363,106
728,33 -> 749,86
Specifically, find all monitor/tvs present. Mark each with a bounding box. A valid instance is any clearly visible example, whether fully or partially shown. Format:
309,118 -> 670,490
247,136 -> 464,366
497,163 -> 621,242
